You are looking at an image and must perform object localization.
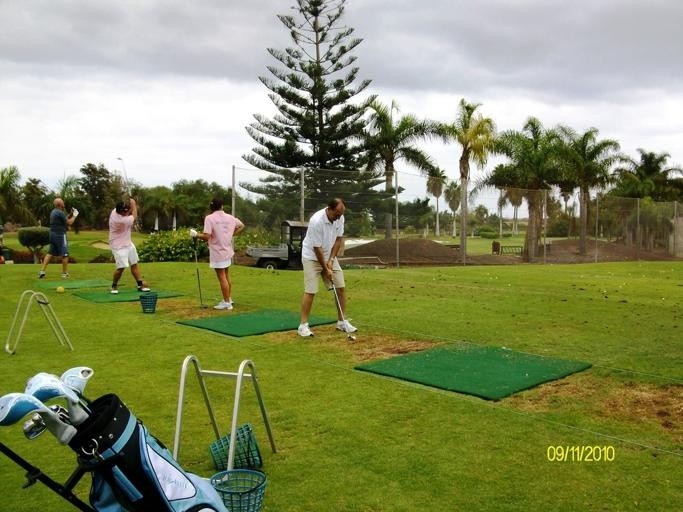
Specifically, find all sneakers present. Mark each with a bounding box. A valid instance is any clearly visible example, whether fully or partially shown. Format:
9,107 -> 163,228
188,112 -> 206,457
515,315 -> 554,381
111,285 -> 119,294
61,273 -> 70,279
214,297 -> 233,310
298,322 -> 313,337
336,320 -> 358,333
137,285 -> 150,291
39,271 -> 45,278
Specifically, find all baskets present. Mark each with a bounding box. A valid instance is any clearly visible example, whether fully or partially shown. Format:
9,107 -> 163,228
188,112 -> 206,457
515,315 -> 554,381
211,469 -> 267,512
209,424 -> 263,470
139,294 -> 157,313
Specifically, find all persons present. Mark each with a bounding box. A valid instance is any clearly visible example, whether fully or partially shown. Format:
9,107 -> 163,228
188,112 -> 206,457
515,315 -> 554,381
186,197 -> 246,311
39,196 -> 80,279
297,196 -> 359,338
108,198 -> 151,294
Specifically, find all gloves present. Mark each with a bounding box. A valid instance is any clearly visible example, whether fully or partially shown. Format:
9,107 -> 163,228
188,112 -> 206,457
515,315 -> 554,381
72,208 -> 78,216
189,229 -> 198,236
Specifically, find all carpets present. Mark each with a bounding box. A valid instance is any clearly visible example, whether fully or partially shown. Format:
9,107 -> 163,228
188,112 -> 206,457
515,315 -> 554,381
74,286 -> 185,304
176,305 -> 337,338
354,338 -> 594,401
35,279 -> 128,290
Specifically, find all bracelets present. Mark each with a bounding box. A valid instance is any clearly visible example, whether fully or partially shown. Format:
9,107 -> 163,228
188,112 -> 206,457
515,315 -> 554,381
329,257 -> 335,262
324,268 -> 328,273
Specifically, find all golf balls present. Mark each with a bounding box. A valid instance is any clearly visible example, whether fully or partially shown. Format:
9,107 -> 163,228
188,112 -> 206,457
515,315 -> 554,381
56,286 -> 65,293
352,336 -> 356,340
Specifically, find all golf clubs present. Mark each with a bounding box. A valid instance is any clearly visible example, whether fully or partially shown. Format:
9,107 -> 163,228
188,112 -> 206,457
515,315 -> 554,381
193,236 -> 208,308
330,278 -> 353,340
0,366 -> 94,446
118,157 -> 132,199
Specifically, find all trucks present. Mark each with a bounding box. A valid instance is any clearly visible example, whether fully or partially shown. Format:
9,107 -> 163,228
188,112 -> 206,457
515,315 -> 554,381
247,220 -> 310,270
